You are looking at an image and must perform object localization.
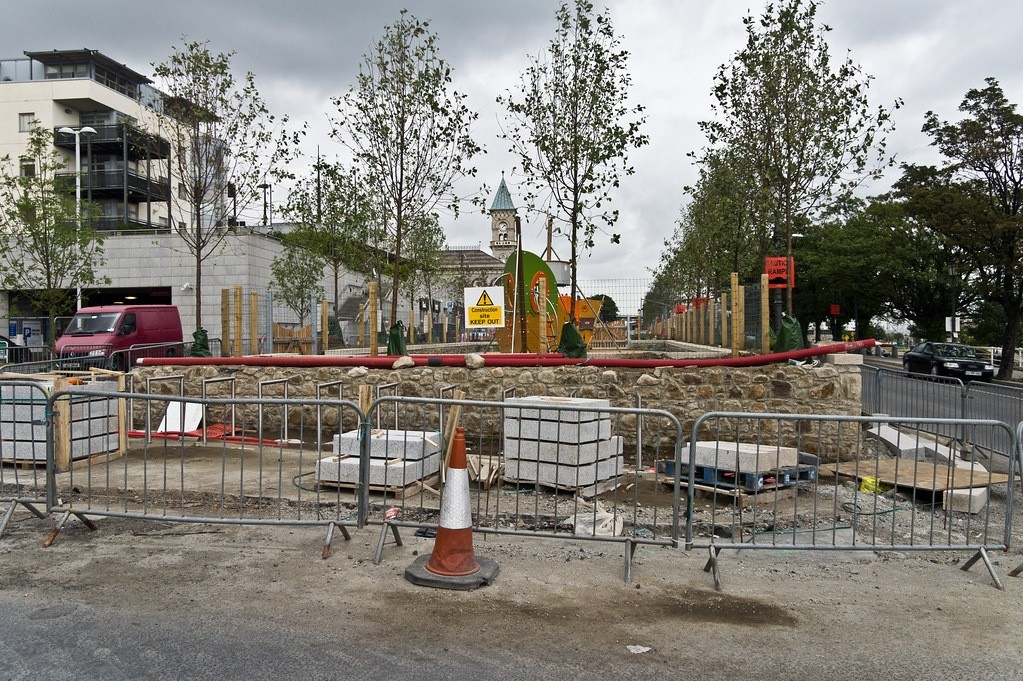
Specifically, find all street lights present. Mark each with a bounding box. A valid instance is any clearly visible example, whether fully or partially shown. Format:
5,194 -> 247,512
257,184 -> 270,225
58,126 -> 99,331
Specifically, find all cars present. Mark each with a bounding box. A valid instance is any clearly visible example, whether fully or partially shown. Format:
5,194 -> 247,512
1,335 -> 33,365
902,341 -> 995,385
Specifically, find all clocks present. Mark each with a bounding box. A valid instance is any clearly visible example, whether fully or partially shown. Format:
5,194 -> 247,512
496,220 -> 510,234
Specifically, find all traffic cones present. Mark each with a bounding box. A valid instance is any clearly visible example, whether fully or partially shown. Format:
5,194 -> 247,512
403,422 -> 501,590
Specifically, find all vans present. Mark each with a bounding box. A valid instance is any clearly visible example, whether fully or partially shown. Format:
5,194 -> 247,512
54,306 -> 184,369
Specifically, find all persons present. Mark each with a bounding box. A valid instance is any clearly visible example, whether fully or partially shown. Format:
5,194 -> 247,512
397,320 -> 416,343
384,321 -> 391,344
417,320 -> 424,343
